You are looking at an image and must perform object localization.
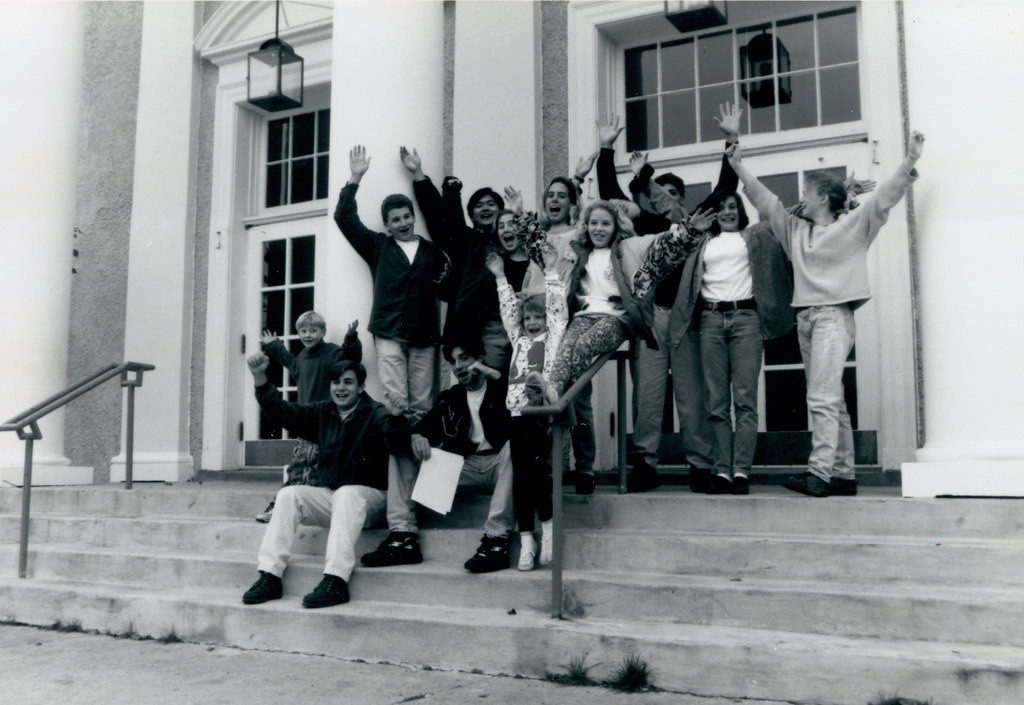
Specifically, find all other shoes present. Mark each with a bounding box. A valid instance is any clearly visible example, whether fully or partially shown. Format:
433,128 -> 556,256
518,540 -> 538,571
576,472 -> 594,494
732,474 -> 748,495
626,462 -> 659,493
540,532 -> 553,565
705,476 -> 733,494
690,464 -> 709,493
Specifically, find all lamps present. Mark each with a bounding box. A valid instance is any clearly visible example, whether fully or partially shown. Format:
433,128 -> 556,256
740,28 -> 792,109
664,0 -> 728,34
246,0 -> 303,112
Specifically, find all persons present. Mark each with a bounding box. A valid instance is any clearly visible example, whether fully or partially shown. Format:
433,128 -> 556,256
488,242 -> 571,571
354,331 -> 517,570
256,312 -> 364,523
500,180 -> 718,406
396,142 -> 600,494
588,101 -> 926,498
328,146 -> 449,414
243,352 -> 414,610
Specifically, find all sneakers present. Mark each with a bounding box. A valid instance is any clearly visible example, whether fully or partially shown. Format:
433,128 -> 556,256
361,530 -> 423,566
524,371 -> 559,407
777,472 -> 830,497
256,501 -> 276,522
303,574 -> 350,608
464,533 -> 512,572
830,477 -> 858,496
243,571 -> 283,604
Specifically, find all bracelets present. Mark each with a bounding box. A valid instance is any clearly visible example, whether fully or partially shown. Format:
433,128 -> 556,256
574,175 -> 585,184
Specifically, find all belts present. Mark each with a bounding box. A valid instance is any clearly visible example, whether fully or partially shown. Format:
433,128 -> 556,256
701,300 -> 758,312
